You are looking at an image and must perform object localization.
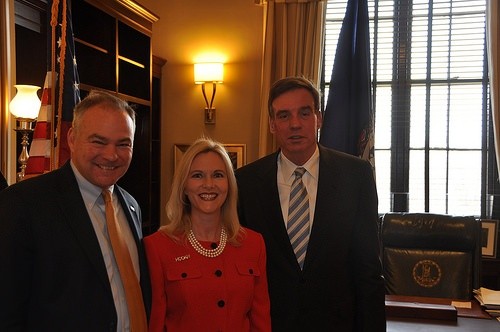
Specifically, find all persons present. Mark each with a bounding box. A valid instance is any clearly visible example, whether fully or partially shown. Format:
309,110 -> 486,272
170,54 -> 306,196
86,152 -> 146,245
0,90 -> 153,332
142,137 -> 271,332
234,77 -> 387,332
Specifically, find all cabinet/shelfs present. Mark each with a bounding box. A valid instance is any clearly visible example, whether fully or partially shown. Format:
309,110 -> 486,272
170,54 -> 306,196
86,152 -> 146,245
10,0 -> 167,237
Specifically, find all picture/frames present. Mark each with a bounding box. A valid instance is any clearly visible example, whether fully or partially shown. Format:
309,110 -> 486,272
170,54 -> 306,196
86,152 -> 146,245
479,218 -> 499,259
172,143 -> 248,179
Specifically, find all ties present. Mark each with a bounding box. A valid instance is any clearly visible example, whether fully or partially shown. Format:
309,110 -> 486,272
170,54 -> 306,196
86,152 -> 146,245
101,189 -> 148,332
287,167 -> 311,270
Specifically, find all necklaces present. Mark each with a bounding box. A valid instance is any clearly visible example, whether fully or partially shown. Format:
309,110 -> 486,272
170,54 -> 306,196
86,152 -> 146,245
187,224 -> 228,258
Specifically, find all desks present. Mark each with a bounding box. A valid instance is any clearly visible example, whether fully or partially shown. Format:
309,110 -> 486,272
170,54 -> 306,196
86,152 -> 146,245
383,294 -> 500,332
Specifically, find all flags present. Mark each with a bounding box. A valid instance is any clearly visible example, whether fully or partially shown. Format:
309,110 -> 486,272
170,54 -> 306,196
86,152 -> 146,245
24,0 -> 82,176
319,0 -> 375,182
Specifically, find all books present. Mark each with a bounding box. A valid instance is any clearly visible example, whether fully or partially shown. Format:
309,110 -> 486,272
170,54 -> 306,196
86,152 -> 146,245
450,287 -> 500,320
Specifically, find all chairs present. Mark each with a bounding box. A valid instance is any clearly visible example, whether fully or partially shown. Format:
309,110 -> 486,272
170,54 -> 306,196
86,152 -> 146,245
378,210 -> 480,298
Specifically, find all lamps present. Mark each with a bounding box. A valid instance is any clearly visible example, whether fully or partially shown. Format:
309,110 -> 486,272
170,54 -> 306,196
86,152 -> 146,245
6,82 -> 43,180
193,62 -> 224,125
